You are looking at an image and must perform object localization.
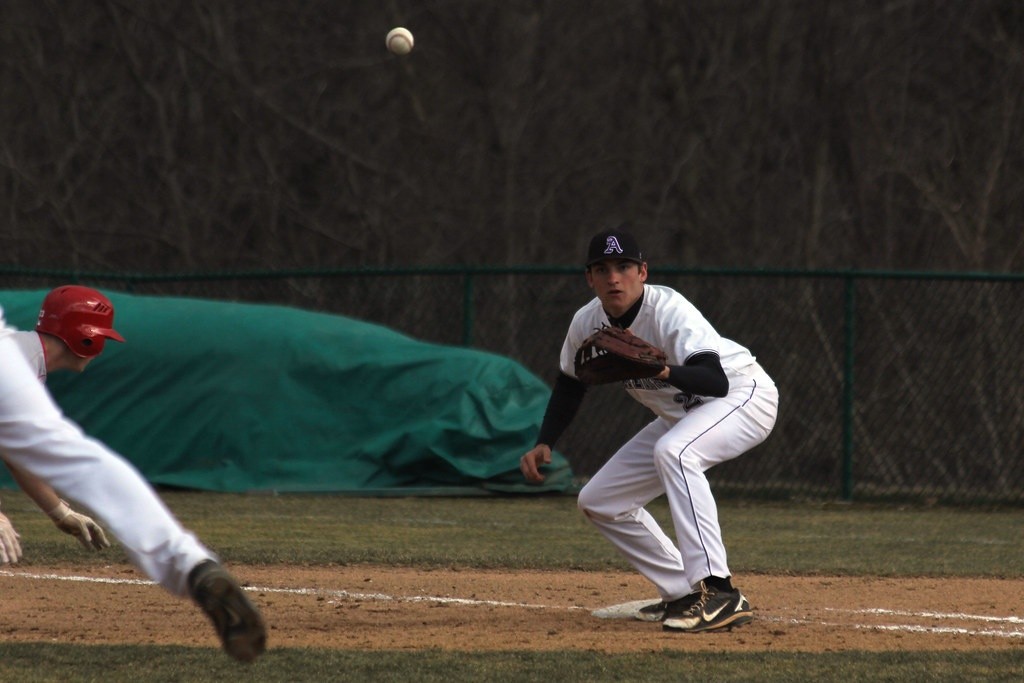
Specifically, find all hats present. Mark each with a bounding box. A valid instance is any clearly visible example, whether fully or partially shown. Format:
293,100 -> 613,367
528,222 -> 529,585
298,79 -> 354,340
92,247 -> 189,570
585,228 -> 643,270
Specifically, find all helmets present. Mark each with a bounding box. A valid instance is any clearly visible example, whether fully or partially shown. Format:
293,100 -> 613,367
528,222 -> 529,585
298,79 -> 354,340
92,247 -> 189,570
36,285 -> 125,358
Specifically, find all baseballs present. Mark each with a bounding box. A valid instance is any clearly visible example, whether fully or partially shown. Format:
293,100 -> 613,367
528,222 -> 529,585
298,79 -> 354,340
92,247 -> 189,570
386,27 -> 414,55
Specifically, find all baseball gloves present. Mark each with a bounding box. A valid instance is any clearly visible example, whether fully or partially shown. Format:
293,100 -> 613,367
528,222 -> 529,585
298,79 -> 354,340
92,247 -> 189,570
573,321 -> 669,386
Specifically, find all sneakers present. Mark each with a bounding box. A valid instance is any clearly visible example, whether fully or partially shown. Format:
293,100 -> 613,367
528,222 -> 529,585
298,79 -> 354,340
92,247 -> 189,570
635,590 -> 702,622
662,580 -> 753,632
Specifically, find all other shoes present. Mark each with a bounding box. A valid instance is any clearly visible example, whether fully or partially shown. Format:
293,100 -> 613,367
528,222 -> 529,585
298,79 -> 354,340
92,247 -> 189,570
187,560 -> 268,661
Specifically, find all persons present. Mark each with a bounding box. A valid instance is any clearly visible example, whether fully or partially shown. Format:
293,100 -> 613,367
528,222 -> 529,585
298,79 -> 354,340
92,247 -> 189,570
520,229 -> 779,630
0,284 -> 127,564
0,306 -> 267,663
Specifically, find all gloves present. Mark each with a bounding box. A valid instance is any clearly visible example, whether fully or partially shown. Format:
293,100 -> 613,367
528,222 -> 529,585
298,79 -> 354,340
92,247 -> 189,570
48,498 -> 111,553
0,512 -> 22,562
